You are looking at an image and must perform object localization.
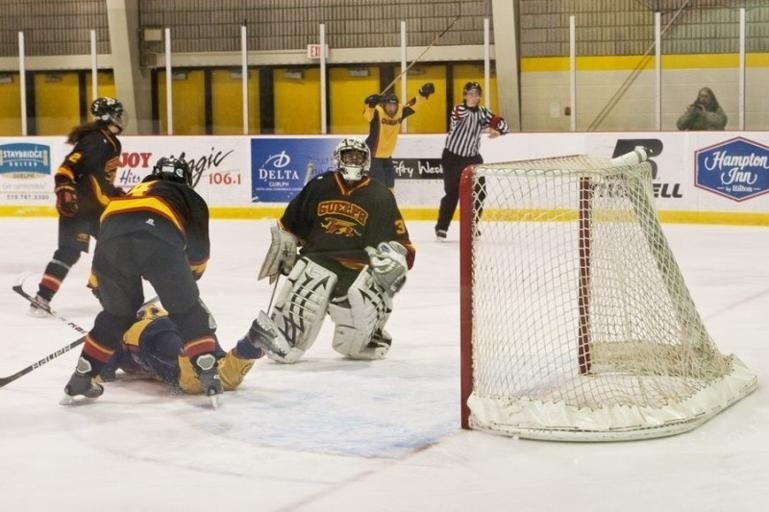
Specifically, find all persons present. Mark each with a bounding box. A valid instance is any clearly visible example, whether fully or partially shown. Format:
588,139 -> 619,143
360,82 -> 435,194
57,154 -> 224,409
258,137 -> 417,364
91,302 -> 293,395
675,86 -> 728,132
433,81 -> 512,242
28,96 -> 127,319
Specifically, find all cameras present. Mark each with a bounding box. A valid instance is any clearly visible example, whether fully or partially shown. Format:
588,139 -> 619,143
692,98 -> 703,109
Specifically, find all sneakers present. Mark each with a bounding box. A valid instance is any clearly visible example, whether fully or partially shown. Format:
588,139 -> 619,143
248,318 -> 287,358
437,228 -> 447,238
32,295 -> 51,312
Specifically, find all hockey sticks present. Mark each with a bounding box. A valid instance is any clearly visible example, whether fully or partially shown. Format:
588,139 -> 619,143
14,271 -> 88,336
381,16 -> 461,96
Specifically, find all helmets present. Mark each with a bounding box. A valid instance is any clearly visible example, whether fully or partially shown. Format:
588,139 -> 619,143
335,138 -> 371,182
464,82 -> 481,95
381,92 -> 399,103
91,97 -> 128,134
153,157 -> 192,187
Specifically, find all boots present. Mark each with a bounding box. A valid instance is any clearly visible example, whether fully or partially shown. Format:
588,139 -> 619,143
191,351 -> 225,395
64,353 -> 105,397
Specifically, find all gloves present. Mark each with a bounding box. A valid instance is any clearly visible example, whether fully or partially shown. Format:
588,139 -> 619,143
419,84 -> 433,98
365,94 -> 381,103
55,185 -> 79,218
